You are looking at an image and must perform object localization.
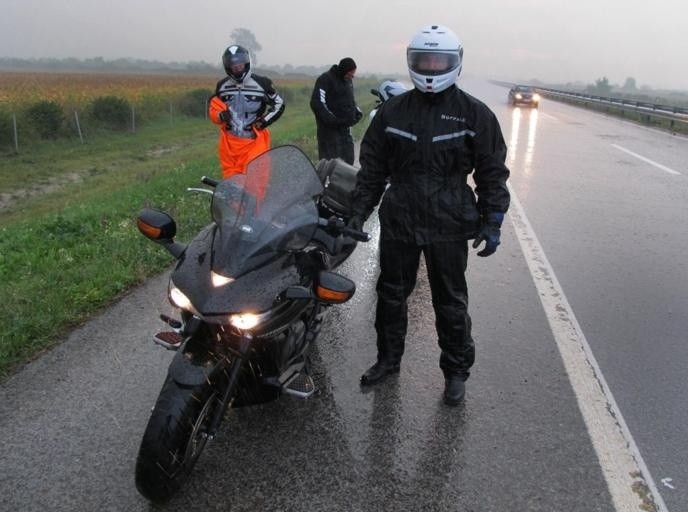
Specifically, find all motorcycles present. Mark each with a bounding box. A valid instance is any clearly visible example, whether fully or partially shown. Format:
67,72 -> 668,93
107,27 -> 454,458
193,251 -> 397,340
369,80 -> 407,122
134,143 -> 369,503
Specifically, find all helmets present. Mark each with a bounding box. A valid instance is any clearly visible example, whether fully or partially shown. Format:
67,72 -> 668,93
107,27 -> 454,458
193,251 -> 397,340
221,44 -> 252,84
370,79 -> 408,109
406,23 -> 464,98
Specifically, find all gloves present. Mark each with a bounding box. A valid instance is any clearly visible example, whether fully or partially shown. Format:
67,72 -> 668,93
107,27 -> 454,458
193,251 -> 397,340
346,207 -> 366,238
472,213 -> 505,257
219,109 -> 233,131
243,118 -> 268,139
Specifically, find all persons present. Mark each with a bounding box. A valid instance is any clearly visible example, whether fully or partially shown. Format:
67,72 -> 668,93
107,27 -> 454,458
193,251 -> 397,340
309,56 -> 363,167
209,43 -> 287,217
345,24 -> 512,405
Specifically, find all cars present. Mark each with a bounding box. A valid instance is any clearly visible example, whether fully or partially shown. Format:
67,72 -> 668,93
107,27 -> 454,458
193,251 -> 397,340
507,84 -> 538,107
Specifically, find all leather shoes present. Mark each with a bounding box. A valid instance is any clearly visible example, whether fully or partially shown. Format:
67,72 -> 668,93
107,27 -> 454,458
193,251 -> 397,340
443,369 -> 467,406
359,356 -> 400,386
